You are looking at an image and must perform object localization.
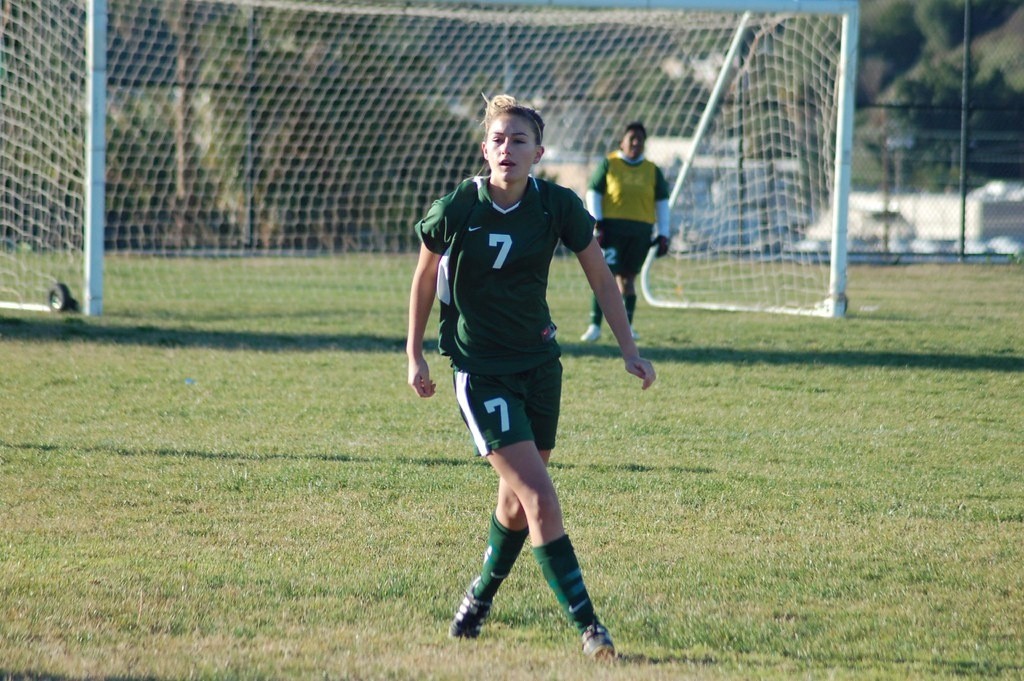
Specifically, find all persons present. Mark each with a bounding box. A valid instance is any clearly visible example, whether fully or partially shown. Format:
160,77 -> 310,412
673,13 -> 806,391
404,95 -> 656,659
580,121 -> 670,341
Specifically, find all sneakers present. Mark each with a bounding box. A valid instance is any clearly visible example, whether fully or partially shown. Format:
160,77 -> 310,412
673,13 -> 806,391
581,624 -> 615,660
581,323 -> 601,342
449,576 -> 492,640
630,325 -> 640,340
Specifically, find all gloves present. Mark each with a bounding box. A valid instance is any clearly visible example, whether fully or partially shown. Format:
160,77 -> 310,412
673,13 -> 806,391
651,235 -> 668,258
596,230 -> 609,248
